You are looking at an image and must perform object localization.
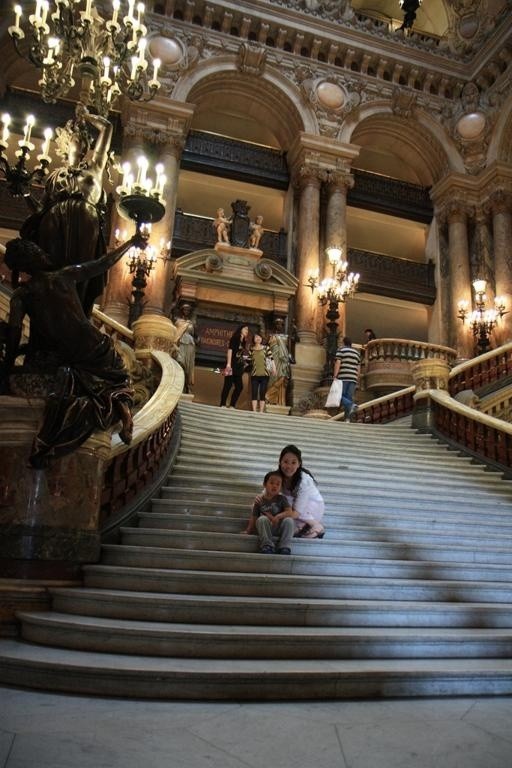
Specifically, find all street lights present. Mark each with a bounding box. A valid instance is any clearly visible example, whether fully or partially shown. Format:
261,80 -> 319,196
111,229 -> 174,326
458,277 -> 508,354
308,244 -> 361,386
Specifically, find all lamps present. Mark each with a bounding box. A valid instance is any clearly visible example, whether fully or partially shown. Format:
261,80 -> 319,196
115,156 -> 168,248
0,112 -> 54,214
8,0 -> 162,130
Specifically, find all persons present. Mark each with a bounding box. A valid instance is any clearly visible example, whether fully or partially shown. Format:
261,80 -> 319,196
248,214 -> 265,249
3,97 -> 115,372
364,328 -> 376,342
211,207 -> 233,242
219,323 -> 250,409
253,444 -> 326,539
245,330 -> 279,414
238,470 -> 295,554
264,318 -> 295,407
1,231 -> 152,446
331,336 -> 362,423
169,298 -> 198,394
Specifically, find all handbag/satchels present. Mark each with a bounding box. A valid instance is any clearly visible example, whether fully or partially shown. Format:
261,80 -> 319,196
264,343 -> 274,373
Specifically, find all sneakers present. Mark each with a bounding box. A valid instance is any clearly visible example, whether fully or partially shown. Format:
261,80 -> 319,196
346,403 -> 359,418
279,545 -> 293,555
260,544 -> 275,553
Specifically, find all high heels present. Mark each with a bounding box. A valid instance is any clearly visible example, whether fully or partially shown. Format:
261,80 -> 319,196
296,523 -> 326,539
